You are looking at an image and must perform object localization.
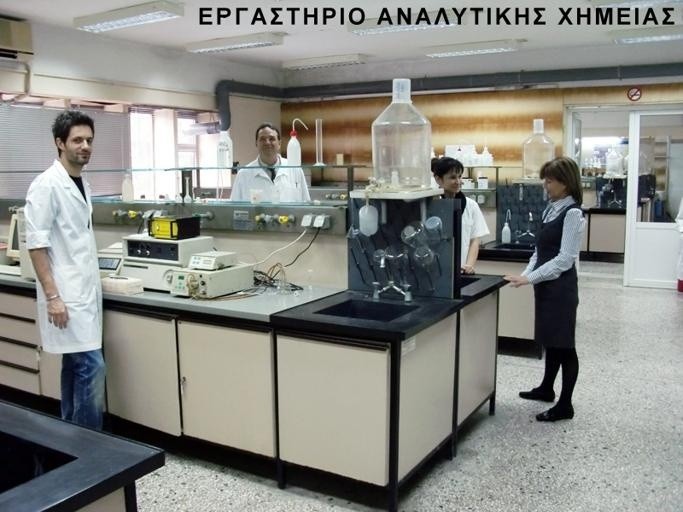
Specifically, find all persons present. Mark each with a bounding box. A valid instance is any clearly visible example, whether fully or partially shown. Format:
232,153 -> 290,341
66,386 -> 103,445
502,155 -> 587,422
431,157 -> 489,274
231,124 -> 309,202
24,111 -> 107,431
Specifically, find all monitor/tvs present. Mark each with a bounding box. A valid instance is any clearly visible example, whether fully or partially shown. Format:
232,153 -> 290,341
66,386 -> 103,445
6,214 -> 21,262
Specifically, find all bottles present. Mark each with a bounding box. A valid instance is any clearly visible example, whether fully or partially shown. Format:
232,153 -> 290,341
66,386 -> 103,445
369,214 -> 443,272
456,148 -> 490,165
120,174 -> 134,202
582,148 -> 652,179
285,131 -> 302,166
501,223 -> 510,244
215,131 -> 233,168
276,271 -> 291,296
174,176 -> 191,204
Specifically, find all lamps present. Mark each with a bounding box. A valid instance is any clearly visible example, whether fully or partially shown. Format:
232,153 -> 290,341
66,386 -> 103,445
184,28 -> 293,56
608,23 -> 682,47
422,37 -> 528,61
278,50 -> 369,73
73,2 -> 185,36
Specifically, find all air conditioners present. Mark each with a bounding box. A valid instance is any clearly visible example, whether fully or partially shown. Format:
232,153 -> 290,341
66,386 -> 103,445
1,14 -> 37,65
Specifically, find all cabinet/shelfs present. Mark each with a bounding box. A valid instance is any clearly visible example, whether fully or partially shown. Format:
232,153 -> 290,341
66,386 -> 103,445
37,297 -> 108,417
104,304 -> 282,460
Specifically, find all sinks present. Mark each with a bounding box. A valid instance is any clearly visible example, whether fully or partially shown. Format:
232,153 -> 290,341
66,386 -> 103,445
310,295 -> 420,324
491,241 -> 537,252
460,274 -> 481,289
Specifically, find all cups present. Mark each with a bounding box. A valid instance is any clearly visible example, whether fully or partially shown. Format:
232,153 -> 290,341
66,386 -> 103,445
248,188 -> 264,204
270,186 -> 281,203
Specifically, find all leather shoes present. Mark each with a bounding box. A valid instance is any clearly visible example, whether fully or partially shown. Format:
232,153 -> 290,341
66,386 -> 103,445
520,387 -> 555,402
536,409 -> 575,422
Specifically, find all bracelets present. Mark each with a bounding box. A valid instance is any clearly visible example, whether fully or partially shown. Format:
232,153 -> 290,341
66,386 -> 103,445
48,294 -> 60,301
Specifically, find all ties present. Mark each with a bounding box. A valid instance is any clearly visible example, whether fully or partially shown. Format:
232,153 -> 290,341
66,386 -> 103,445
269,167 -> 276,180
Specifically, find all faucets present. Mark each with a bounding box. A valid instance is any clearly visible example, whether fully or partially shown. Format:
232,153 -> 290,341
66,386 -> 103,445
516,211 -> 536,239
372,245 -> 404,270
